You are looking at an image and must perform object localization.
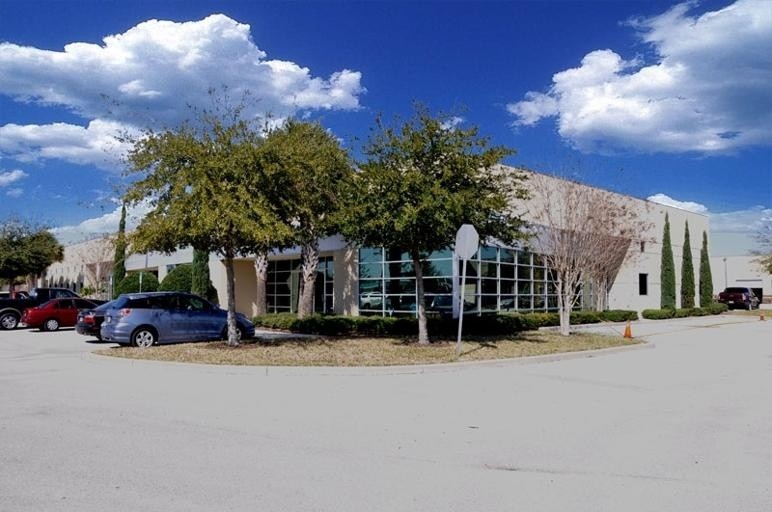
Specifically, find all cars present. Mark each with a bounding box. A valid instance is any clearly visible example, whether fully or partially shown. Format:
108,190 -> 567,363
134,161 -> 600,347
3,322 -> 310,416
359,291 -> 392,310
0,287 -> 256,348
718,287 -> 760,312
411,293 -> 480,320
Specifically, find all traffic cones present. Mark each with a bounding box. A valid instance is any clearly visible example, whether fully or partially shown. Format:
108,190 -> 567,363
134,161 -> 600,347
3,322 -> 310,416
757,309 -> 767,322
623,319 -> 635,339
